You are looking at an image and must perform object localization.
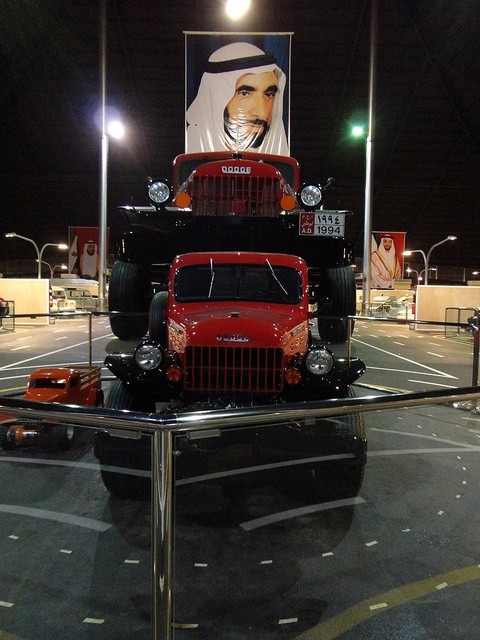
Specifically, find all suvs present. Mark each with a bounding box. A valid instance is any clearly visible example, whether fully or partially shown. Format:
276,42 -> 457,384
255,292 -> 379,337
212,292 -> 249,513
93,252 -> 368,499
108,150 -> 352,338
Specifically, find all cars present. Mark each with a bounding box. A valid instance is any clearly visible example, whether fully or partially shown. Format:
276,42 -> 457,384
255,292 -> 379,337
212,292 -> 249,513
369,296 -> 410,317
397,304 -> 415,322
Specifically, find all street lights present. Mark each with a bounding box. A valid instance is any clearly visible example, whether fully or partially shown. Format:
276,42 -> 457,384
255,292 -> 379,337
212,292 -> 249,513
5,232 -> 68,278
403,235 -> 456,285
34,259 -> 67,278
405,268 -> 436,284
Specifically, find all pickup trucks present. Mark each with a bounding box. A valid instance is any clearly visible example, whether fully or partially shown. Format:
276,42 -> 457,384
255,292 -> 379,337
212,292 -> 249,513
49,285 -> 75,311
63,287 -> 107,315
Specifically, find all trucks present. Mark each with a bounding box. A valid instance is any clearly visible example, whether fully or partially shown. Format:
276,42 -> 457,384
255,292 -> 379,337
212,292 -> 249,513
368,296 -> 388,316
0,365 -> 104,452
